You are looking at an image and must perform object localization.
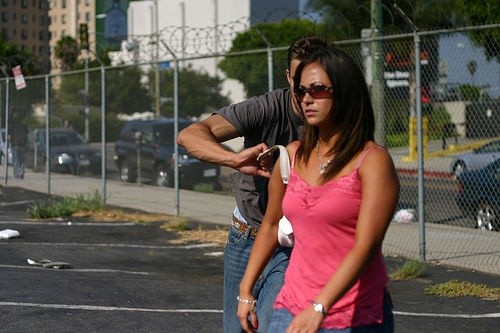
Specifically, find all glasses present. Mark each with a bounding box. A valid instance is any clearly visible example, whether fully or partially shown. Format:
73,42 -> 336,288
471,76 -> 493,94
295,84 -> 334,102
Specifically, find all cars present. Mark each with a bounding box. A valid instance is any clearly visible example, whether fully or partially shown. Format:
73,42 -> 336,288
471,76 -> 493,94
450,138 -> 500,232
0,128 -> 13,165
27,127 -> 102,175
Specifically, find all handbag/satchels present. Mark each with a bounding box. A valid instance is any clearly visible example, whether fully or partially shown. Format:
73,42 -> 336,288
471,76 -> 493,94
257,144 -> 294,248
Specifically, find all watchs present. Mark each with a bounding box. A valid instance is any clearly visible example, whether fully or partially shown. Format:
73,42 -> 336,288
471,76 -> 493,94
309,300 -> 327,318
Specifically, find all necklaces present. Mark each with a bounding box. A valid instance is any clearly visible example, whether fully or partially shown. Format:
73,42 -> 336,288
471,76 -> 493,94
315,141 -> 336,175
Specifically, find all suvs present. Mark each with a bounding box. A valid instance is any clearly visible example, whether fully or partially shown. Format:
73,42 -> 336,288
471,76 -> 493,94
113,120 -> 221,190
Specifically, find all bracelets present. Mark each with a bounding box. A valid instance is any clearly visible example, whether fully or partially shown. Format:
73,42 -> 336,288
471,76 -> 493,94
235,296 -> 259,305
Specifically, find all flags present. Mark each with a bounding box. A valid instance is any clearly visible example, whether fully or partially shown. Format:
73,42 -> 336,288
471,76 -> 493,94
12,64 -> 26,90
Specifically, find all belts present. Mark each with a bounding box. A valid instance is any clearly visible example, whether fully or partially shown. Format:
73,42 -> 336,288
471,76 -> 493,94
232,215 -> 258,237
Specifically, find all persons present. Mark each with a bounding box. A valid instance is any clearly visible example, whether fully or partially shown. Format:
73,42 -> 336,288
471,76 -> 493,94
235,47 -> 403,333
64,119 -> 72,129
10,111 -> 29,180
176,34 -> 331,333
